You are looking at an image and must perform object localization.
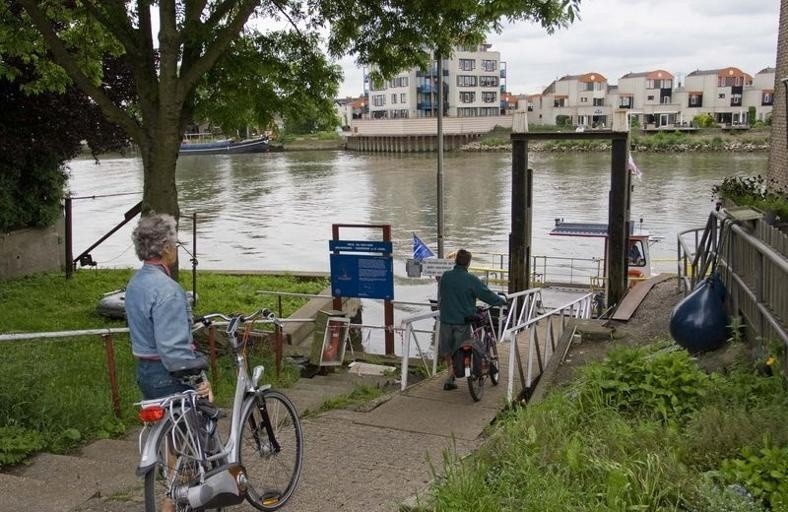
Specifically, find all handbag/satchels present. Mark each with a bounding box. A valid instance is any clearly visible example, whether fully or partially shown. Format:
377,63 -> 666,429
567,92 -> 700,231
451,338 -> 499,378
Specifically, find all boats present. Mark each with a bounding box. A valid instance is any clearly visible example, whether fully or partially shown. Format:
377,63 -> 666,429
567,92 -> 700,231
178,132 -> 270,155
550,215 -> 636,239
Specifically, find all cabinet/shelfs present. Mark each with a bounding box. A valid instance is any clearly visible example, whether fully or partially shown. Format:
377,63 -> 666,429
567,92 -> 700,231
309,308 -> 351,366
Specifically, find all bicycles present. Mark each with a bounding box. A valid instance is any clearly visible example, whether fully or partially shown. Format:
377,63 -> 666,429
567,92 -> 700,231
132,307 -> 304,512
457,293 -> 510,402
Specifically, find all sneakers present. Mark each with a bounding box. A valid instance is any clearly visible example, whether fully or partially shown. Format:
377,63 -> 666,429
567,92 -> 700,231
444,383 -> 457,391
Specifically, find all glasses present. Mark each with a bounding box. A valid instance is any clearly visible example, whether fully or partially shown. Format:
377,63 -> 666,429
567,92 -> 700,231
162,240 -> 181,248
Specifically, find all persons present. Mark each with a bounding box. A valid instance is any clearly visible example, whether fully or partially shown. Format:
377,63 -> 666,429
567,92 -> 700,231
627,238 -> 642,266
437,248 -> 507,392
124,211 -> 225,512
